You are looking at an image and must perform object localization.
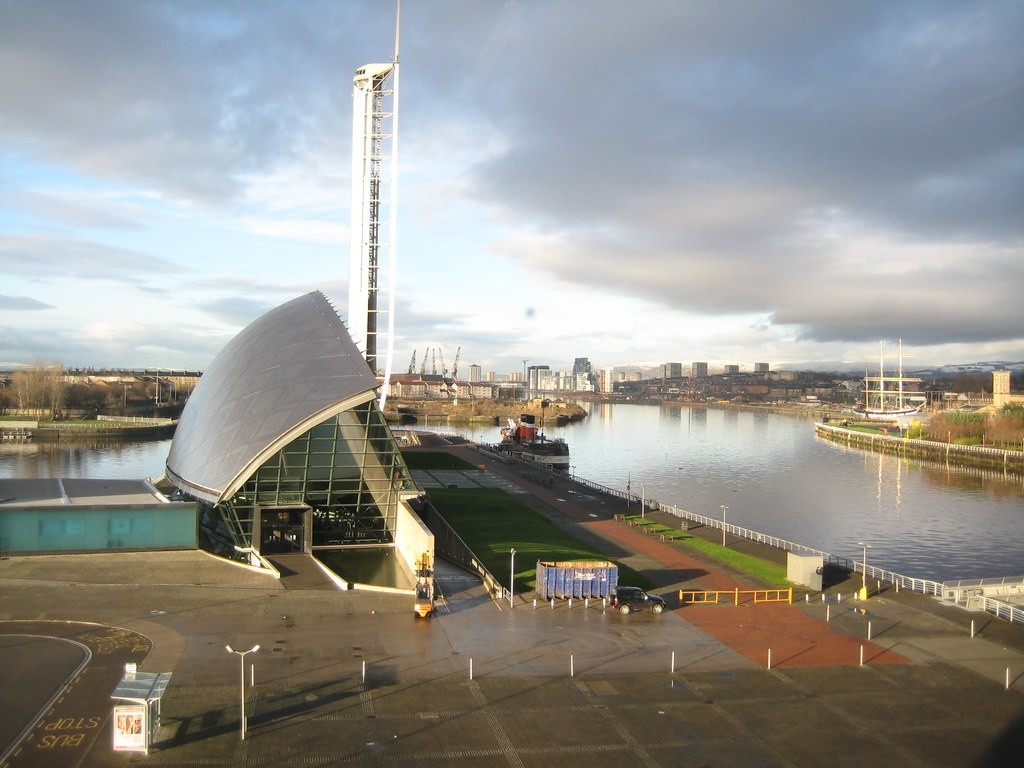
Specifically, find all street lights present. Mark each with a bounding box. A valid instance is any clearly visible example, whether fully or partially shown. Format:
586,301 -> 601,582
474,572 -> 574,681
641,483 -> 648,519
720,505 -> 729,548
510,546 -> 517,609
856,540 -> 872,588
224,644 -> 262,742
571,466 -> 576,496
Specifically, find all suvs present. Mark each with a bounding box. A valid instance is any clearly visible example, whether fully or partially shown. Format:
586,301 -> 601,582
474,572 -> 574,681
608,586 -> 666,616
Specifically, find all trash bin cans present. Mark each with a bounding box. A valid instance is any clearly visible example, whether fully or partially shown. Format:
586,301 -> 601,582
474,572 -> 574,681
649,497 -> 656,511
680,520 -> 689,532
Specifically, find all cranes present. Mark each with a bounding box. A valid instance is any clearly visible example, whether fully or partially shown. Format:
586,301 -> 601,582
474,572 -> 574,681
408,346 -> 462,382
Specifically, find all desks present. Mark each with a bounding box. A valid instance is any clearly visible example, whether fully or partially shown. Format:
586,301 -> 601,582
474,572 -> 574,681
642,526 -> 656,536
628,520 -> 640,528
660,533 -> 673,544
614,514 -> 625,521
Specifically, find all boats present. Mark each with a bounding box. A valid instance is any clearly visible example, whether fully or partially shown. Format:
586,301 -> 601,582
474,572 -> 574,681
499,393 -> 570,470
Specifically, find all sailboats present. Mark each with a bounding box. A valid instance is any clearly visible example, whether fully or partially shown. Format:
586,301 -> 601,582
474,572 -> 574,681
851,336 -> 928,421
